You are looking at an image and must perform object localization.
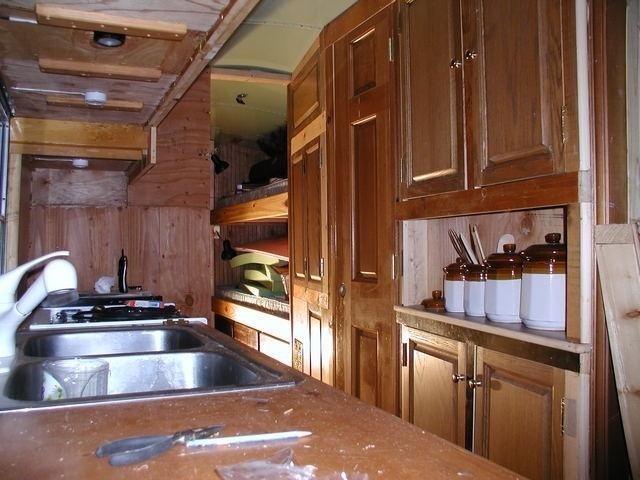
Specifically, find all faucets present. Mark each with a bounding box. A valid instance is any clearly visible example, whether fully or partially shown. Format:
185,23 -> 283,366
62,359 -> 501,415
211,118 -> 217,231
0,251 -> 78,355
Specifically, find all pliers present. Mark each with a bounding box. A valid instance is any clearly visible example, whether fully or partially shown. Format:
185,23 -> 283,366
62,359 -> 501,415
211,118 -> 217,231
95,425 -> 223,468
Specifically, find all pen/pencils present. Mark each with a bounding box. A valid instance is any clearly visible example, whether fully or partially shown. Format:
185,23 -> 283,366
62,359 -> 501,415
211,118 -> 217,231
186,430 -> 314,446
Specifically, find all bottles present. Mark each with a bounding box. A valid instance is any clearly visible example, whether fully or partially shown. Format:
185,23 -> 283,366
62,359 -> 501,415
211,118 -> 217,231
442,232 -> 566,330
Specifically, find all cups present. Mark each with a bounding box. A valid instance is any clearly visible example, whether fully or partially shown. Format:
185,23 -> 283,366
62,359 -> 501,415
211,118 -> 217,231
40,358 -> 110,400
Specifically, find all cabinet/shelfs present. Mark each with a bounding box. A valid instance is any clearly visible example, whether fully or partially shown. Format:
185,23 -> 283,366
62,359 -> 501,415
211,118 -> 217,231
400,0 -> 577,199
398,202 -> 577,348
288,1 -> 397,416
230,320 -> 289,366
397,324 -> 565,480
210,191 -> 291,345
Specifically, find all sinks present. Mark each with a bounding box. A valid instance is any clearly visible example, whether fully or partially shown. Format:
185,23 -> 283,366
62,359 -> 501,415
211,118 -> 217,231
0,355 -> 309,410
15,323 -> 211,357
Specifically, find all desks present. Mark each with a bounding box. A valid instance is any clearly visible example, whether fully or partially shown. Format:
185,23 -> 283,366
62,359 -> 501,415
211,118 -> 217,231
0,321 -> 529,480
